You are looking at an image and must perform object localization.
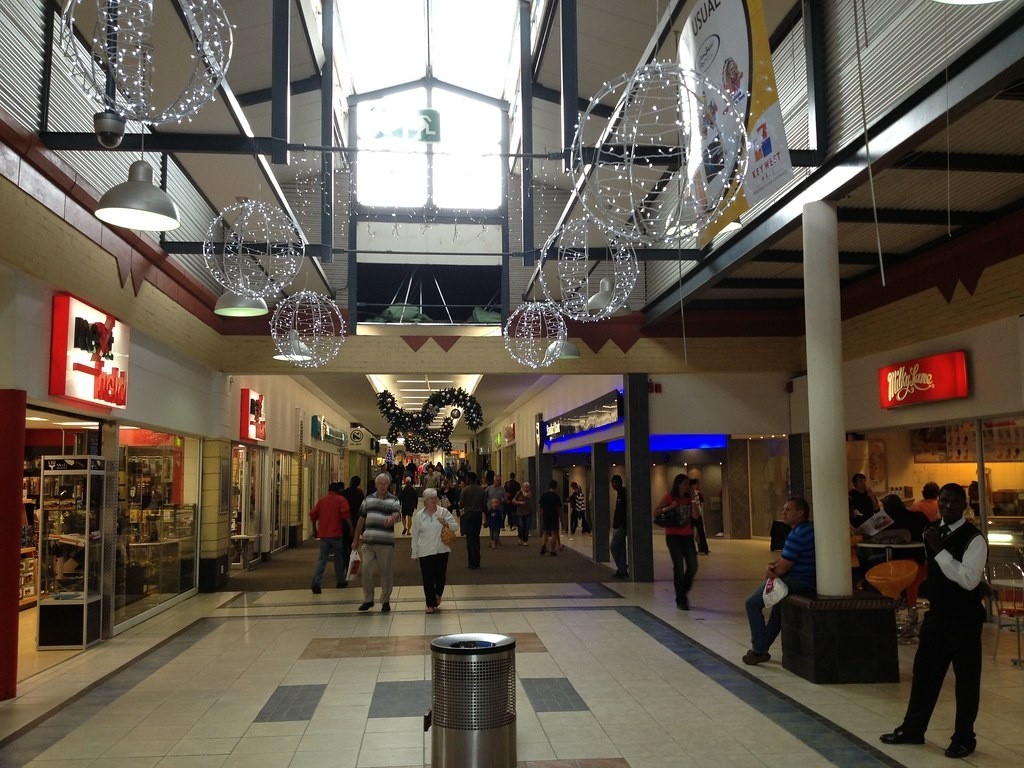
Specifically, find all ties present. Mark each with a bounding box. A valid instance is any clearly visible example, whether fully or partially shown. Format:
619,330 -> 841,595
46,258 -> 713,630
939,524 -> 950,536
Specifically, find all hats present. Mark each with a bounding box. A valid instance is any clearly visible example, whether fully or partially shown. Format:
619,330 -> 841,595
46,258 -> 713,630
406,477 -> 411,483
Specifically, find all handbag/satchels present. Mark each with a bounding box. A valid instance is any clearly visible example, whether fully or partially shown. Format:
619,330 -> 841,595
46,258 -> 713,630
346,550 -> 361,581
652,497 -> 683,527
762,571 -> 789,608
440,508 -> 457,545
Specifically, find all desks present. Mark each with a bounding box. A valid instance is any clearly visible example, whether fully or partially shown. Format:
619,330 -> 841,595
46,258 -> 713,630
856,542 -> 924,562
991,579 -> 1024,588
231,534 -> 263,571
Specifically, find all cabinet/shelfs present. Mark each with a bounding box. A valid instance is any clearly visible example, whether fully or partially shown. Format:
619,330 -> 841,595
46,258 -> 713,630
20,455 -> 172,652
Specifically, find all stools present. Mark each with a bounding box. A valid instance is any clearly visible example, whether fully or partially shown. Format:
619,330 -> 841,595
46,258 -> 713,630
864,559 -> 930,646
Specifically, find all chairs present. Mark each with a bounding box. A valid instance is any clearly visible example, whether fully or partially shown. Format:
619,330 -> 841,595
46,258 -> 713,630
983,561 -> 1024,670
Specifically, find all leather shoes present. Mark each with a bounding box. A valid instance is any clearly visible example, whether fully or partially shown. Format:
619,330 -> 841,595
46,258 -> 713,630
880,727 -> 925,745
945,739 -> 976,757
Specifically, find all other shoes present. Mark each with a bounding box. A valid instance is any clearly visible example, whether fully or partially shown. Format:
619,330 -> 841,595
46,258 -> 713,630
492,540 -> 496,549
677,603 -> 689,610
489,541 -> 492,547
310,583 -> 321,594
540,545 -> 547,554
381,602 -> 391,612
358,601 -> 374,611
698,550 -> 710,554
496,541 -> 501,546
407,533 -> 411,535
402,528 -> 407,534
426,607 -> 435,613
524,542 -> 528,545
336,582 -> 348,588
436,594 -> 441,607
550,551 -> 557,556
742,650 -> 771,665
518,538 -> 522,545
611,572 -> 629,580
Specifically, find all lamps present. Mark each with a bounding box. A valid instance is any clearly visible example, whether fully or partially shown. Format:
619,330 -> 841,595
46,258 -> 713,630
213,196 -> 270,317
272,272 -> 313,361
546,275 -> 582,359
667,30 -> 708,237
92,43 -> 182,231
583,202 -> 632,317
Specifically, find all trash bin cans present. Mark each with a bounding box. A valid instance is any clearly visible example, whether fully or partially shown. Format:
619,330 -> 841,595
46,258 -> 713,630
429,632 -> 518,768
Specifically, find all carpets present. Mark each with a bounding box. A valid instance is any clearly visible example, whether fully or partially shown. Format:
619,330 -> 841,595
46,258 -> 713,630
220,536 -> 632,591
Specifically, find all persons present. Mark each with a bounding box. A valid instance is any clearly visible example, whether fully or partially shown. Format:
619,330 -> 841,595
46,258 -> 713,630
848,473 -> 880,528
458,472 -> 487,570
879,483 -> 991,760
409,488 -> 459,613
369,459 -> 521,535
909,481 -> 942,522
610,475 -> 629,577
968,481 -> 980,516
653,474 -> 700,610
881,494 -> 930,564
512,482 -> 533,546
850,524 -> 863,590
486,499 -> 503,549
689,479 -> 711,554
309,481 -> 354,594
742,497 -> 816,665
351,473 -> 400,611
538,480 -> 590,557
484,475 -> 507,546
344,475 -> 365,535
